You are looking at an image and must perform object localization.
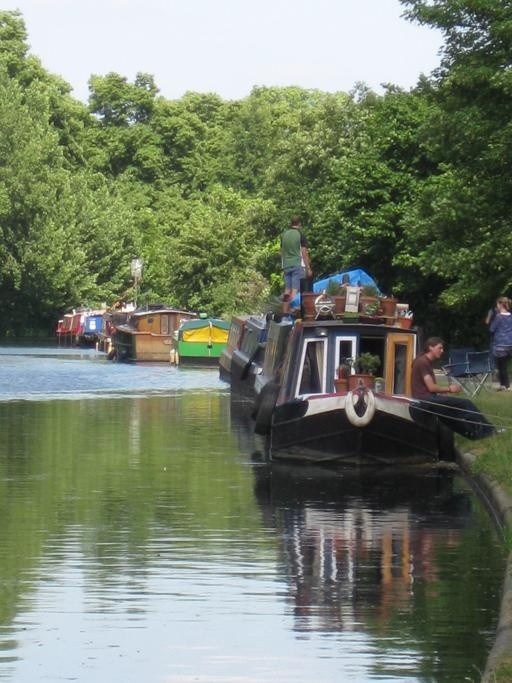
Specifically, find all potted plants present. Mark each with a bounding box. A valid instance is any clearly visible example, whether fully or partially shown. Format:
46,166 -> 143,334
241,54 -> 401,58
334,353 -> 385,394
303,280 -> 414,330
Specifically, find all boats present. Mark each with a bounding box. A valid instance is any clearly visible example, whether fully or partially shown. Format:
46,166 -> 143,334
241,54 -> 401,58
242,463 -> 473,645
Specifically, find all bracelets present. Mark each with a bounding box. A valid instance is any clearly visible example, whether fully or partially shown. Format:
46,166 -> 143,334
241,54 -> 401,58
306,264 -> 310,268
447,386 -> 451,392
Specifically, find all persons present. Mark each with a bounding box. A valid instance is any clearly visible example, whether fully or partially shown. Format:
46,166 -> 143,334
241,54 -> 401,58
486,296 -> 512,393
278,214 -> 312,317
408,336 -> 505,441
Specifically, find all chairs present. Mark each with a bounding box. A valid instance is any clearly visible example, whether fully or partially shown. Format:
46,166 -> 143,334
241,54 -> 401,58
439,346 -> 493,402
